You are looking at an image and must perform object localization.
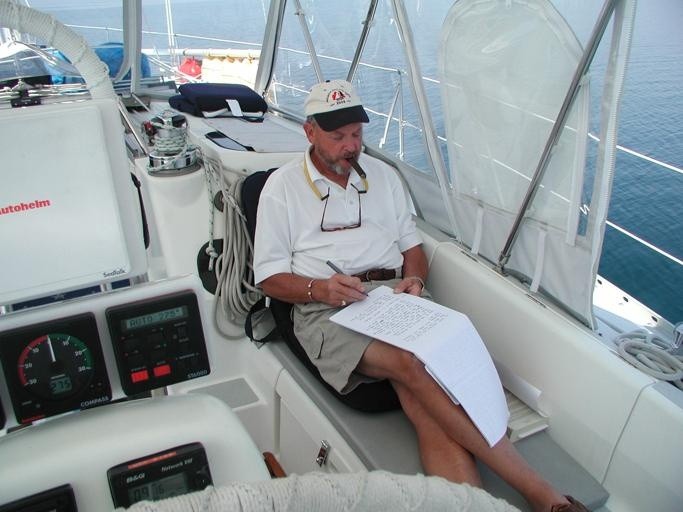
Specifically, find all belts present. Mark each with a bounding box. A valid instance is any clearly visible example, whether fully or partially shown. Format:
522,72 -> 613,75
352,266 -> 403,283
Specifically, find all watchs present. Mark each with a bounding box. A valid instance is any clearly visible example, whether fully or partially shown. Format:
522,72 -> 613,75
405,275 -> 425,296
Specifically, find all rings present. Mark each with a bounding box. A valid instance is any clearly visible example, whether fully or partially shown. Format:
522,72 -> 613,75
341,300 -> 347,309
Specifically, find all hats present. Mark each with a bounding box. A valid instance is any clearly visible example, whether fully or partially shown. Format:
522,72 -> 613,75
306,79 -> 370,131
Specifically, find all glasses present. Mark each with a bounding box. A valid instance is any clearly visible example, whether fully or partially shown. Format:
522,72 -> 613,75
320,183 -> 363,231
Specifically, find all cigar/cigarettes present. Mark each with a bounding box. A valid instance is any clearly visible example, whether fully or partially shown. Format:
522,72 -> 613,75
347,157 -> 367,180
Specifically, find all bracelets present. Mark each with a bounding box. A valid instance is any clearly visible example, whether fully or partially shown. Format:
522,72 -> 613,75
308,277 -> 316,302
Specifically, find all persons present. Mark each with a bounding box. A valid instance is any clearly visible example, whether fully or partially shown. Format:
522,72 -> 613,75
253,79 -> 590,511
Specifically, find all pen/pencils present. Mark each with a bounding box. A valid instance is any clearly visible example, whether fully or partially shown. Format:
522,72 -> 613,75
326,260 -> 369,296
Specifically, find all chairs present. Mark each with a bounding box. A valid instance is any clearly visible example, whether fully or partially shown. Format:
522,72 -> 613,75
241,168 -> 411,411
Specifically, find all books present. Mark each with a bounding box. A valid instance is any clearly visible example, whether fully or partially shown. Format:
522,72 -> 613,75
329,284 -> 511,449
493,358 -> 550,444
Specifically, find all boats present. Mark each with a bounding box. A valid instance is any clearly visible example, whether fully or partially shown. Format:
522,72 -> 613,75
0,0 -> 683,512
